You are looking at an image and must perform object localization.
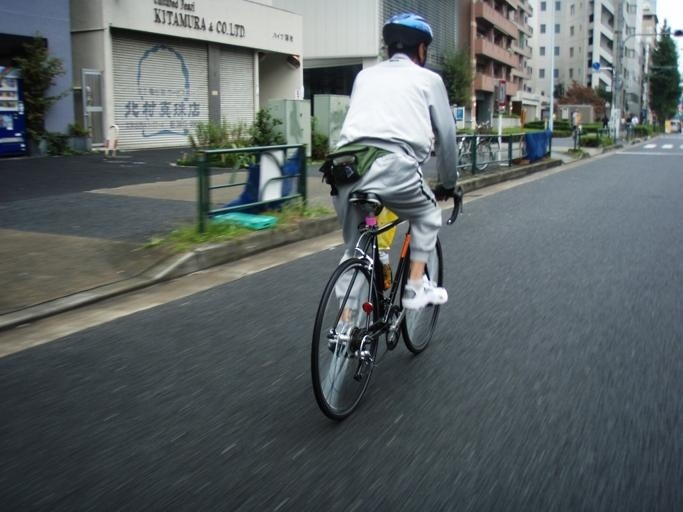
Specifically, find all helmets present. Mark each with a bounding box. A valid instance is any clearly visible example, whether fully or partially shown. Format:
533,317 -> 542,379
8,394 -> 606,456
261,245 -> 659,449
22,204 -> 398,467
382,11 -> 433,47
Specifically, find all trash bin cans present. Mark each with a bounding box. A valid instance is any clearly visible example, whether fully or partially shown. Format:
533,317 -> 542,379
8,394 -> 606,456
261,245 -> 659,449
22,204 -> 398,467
665,121 -> 671,133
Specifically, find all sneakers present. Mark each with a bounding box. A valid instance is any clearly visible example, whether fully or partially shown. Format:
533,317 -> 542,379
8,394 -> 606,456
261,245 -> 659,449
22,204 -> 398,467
401,273 -> 448,310
328,320 -> 374,351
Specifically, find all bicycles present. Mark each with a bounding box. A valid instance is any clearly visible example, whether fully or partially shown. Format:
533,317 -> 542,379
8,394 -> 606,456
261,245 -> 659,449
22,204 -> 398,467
309,183 -> 464,421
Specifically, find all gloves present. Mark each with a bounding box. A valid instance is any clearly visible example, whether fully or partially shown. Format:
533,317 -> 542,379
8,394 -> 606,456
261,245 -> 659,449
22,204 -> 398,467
435,183 -> 455,201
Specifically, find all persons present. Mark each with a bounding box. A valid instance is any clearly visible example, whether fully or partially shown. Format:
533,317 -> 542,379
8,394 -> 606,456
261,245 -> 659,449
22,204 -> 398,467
624,113 -> 640,134
571,109 -> 579,137
329,13 -> 457,359
602,115 -> 609,129
